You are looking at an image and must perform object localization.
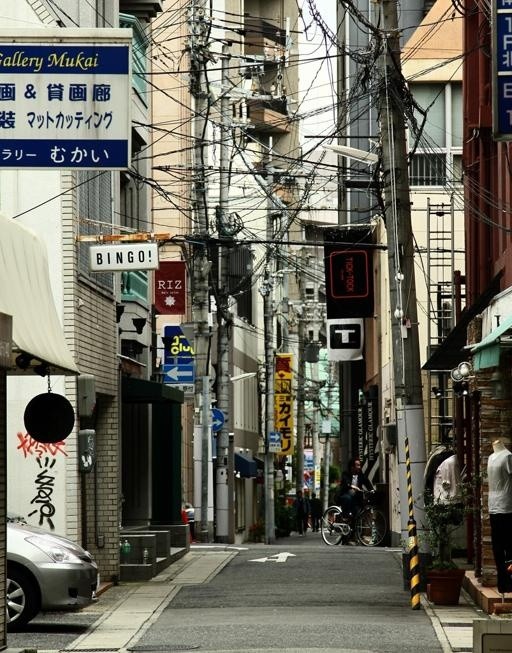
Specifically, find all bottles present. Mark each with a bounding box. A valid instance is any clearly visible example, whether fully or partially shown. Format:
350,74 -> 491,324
119,540 -> 131,564
143,548 -> 149,564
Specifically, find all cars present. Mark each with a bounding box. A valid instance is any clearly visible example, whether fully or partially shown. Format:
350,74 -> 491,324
6,518 -> 101,634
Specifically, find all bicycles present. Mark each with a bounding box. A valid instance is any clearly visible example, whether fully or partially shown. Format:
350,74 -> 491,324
320,486 -> 389,546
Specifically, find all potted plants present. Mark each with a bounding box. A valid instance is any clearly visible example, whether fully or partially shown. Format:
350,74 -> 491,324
411,469 -> 487,605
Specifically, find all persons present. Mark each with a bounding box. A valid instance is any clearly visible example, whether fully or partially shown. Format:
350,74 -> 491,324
308,492 -> 323,532
332,456 -> 376,547
292,490 -> 310,536
425,444 -> 462,525
486,438 -> 511,595
